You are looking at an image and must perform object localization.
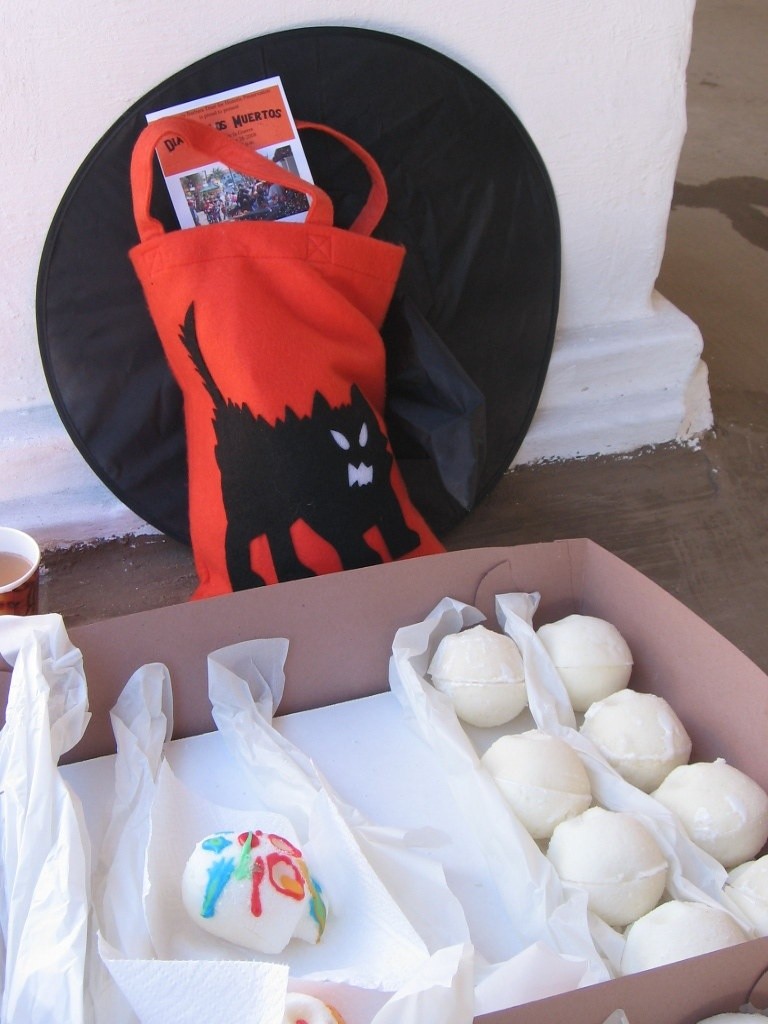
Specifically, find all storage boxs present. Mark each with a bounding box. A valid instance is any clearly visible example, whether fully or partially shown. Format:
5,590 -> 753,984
0,534 -> 768,1024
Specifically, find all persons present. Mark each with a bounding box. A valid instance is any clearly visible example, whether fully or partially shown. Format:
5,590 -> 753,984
184,175 -> 308,227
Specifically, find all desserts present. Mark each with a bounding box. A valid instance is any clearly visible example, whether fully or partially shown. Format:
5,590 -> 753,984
426,615 -> 768,979
180,830 -> 347,1024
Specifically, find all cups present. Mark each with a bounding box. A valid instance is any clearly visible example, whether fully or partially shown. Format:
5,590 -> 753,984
1,523 -> 40,615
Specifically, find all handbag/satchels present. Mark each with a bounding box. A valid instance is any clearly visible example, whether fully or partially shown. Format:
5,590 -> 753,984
127,117 -> 444,598
